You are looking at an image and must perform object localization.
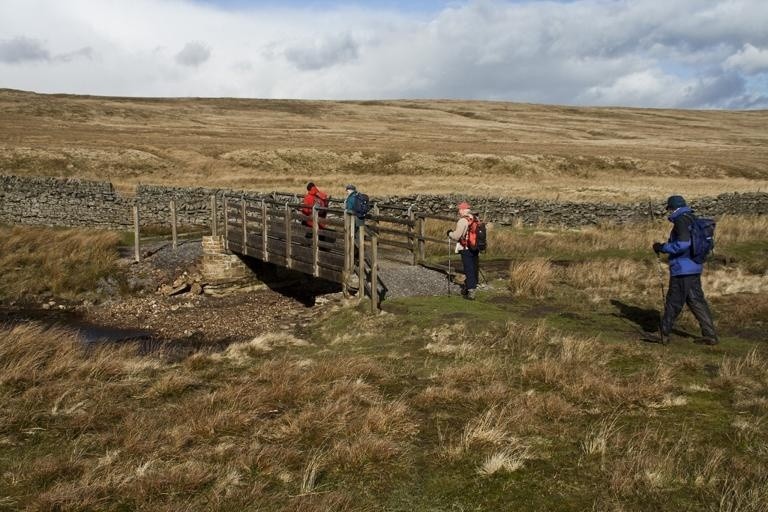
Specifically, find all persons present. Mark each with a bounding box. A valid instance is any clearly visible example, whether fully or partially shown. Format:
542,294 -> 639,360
300,183 -> 330,251
641,196 -> 718,345
446,201 -> 479,299
343,185 -> 370,261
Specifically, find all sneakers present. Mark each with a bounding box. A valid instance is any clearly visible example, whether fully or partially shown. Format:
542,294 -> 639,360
695,334 -> 718,346
461,288 -> 477,300
645,332 -> 668,345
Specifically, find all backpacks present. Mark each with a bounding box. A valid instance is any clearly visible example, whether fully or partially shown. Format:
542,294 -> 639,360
685,213 -> 715,264
354,193 -> 370,220
460,216 -> 487,252
313,196 -> 328,217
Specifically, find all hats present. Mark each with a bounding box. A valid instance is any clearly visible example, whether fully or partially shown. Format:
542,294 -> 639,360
346,185 -> 355,190
666,196 -> 686,210
306,182 -> 314,190
458,203 -> 470,209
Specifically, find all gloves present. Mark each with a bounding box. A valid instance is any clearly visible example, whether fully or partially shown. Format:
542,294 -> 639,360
447,228 -> 452,236
653,243 -> 664,252
